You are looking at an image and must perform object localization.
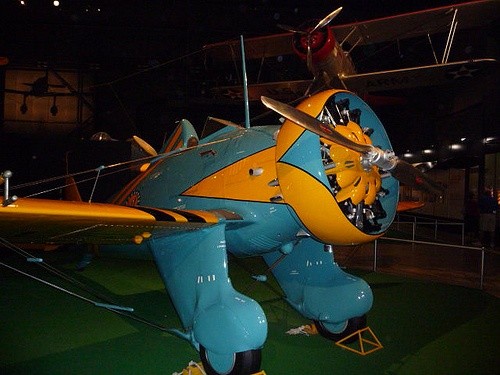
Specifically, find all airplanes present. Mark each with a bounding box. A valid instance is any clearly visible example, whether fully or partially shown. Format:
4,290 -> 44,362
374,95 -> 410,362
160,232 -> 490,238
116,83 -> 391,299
0,87 -> 447,375
202,1 -> 500,103
